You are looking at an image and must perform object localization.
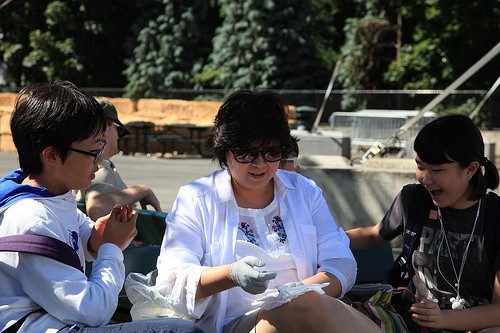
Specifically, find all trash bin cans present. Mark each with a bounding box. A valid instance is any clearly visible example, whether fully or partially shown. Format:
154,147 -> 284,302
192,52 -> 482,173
295,105 -> 319,134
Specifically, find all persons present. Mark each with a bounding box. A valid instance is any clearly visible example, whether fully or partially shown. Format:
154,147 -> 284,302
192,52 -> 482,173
157,88 -> 383,333
346,114 -> 500,333
0,78 -> 205,333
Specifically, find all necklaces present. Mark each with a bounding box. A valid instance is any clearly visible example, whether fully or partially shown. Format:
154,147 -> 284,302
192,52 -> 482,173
435,197 -> 481,311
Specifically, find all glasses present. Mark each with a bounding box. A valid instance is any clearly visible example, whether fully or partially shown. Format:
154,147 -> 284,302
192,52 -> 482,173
110,121 -> 125,135
226,146 -> 292,164
56,139 -> 110,166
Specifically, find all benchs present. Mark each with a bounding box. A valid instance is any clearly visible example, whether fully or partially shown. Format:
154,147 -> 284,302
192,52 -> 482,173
157,133 -> 181,158
79,204 -> 393,326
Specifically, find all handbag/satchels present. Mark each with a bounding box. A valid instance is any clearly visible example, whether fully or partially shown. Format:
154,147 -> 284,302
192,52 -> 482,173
382,254 -> 416,288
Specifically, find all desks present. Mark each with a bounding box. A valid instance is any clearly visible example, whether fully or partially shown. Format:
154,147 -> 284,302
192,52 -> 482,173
126,120 -> 209,158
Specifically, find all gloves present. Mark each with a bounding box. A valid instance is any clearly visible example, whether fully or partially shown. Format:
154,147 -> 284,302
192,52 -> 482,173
276,281 -> 305,294
230,255 -> 278,295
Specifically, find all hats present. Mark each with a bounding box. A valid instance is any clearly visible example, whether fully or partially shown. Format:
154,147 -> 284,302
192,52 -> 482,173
95,99 -> 130,137
